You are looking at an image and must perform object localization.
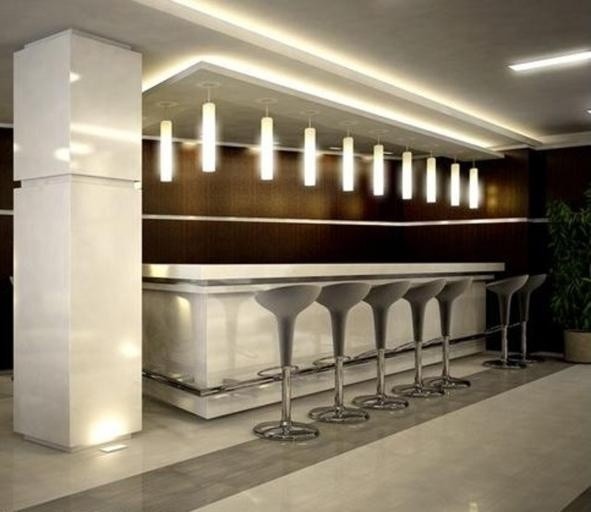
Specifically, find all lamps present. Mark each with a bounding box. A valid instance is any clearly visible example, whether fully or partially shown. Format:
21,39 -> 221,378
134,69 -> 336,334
342,126 -> 353,192
373,133 -> 385,196
303,116 -> 316,187
469,156 -> 479,209
201,88 -> 216,174
159,101 -> 173,183
260,98 -> 274,181
426,149 -> 436,203
401,143 -> 412,200
450,156 -> 460,206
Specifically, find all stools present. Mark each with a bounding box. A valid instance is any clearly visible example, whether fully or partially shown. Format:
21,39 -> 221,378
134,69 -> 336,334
351,280 -> 415,412
485,274 -> 526,369
253,283 -> 322,440
391,279 -> 447,400
517,274 -> 546,369
421,277 -> 472,391
308,282 -> 373,423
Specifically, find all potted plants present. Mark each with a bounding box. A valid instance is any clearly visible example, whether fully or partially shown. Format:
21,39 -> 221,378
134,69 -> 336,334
545,194 -> 591,366
